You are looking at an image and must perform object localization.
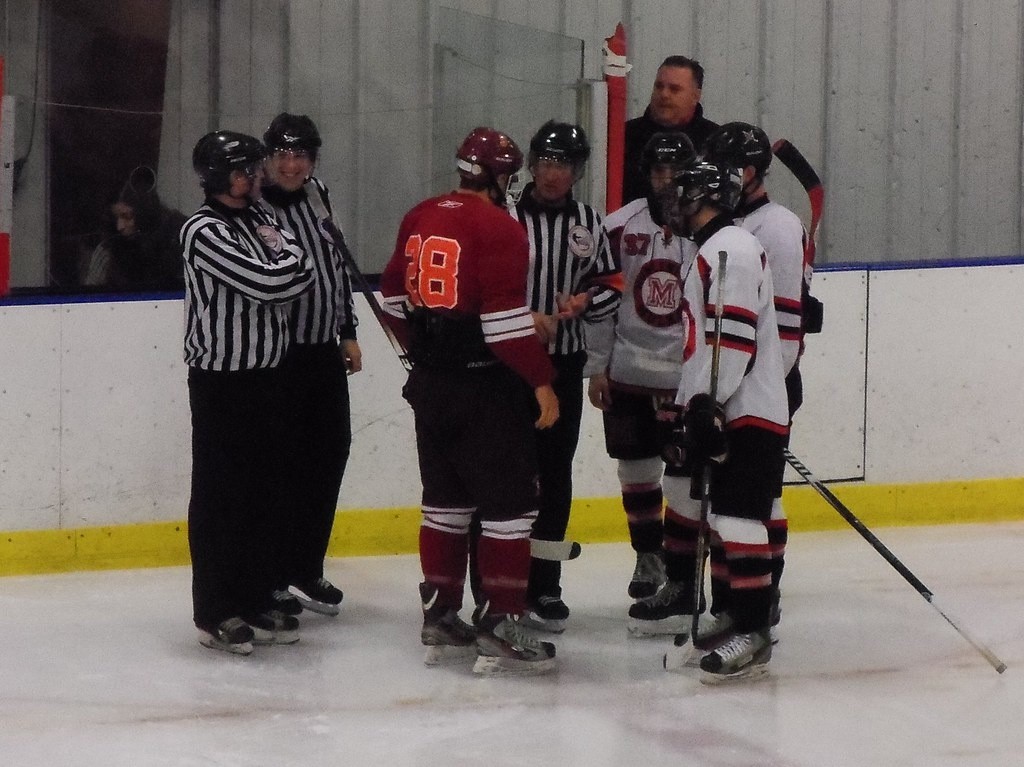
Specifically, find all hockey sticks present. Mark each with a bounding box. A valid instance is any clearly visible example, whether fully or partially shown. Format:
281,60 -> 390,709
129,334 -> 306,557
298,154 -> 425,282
663,251 -> 728,670
783,447 -> 1009,675
772,137 -> 826,367
301,174 -> 582,562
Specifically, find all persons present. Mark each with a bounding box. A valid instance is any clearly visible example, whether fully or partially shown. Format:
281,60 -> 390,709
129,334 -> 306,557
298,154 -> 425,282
380,126 -> 559,660
624,56 -> 721,204
582,130 -> 693,598
690,122 -> 808,634
257,113 -> 362,616
181,130 -> 316,644
648,158 -> 789,674
515,118 -> 625,620
79,173 -> 189,291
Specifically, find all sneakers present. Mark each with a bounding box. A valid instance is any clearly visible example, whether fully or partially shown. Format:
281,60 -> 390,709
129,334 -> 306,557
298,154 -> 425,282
415,579 -> 478,669
516,584 -> 571,631
699,626 -> 775,687
625,581 -> 710,636
627,551 -> 668,603
265,582 -> 306,620
685,589 -> 784,667
285,575 -> 347,615
194,613 -> 256,657
244,606 -> 302,645
468,603 -> 557,675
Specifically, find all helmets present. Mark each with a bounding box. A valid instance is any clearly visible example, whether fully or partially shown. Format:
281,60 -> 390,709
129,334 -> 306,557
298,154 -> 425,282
705,120 -> 773,171
456,124 -> 523,184
262,112 -> 326,153
526,119 -> 596,160
191,128 -> 267,189
674,146 -> 745,215
641,130 -> 696,169
